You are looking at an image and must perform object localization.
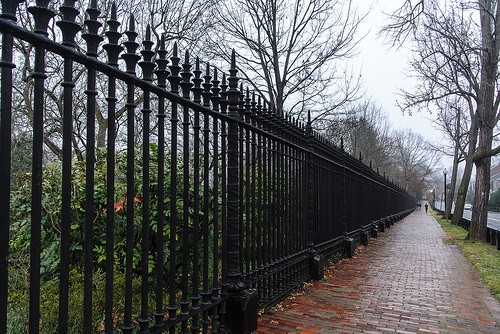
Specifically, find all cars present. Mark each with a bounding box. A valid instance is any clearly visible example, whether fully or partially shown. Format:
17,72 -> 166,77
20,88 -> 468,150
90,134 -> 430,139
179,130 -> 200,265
465,203 -> 471,209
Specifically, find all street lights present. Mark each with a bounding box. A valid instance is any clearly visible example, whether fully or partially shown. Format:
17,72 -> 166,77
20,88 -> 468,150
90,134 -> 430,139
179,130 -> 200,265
427,187 -> 435,210
443,168 -> 449,210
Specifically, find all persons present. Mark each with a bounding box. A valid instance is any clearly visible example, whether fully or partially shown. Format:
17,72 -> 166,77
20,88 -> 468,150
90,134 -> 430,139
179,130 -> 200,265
425,203 -> 428,212
